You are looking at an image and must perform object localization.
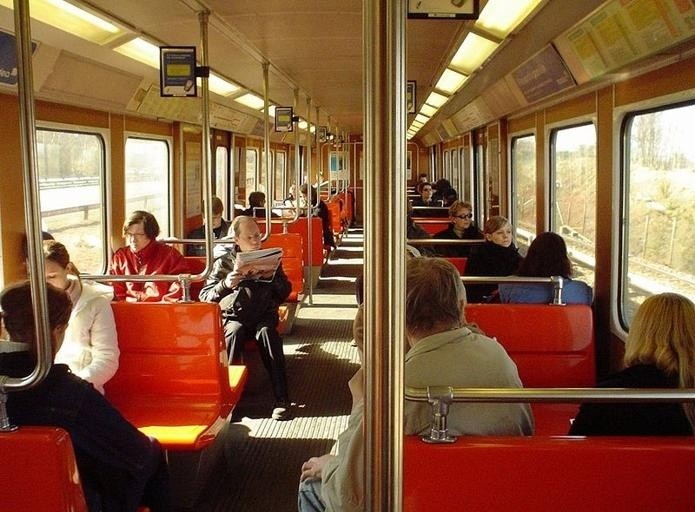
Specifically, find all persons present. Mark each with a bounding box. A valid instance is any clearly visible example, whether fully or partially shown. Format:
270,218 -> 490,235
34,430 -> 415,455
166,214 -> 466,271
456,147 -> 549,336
186,196 -> 229,256
353,167 -> 593,354
21,231 -> 118,301
199,212 -> 292,423
0,278 -> 186,512
41,238 -> 121,406
239,192 -> 282,218
296,258 -> 534,510
313,171 -> 330,188
570,291 -> 695,436
106,209 -> 195,304
300,184 -> 338,250
284,182 -> 303,209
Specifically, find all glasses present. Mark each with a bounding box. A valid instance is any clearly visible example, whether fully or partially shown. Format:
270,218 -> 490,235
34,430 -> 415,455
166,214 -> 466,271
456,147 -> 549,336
452,214 -> 472,218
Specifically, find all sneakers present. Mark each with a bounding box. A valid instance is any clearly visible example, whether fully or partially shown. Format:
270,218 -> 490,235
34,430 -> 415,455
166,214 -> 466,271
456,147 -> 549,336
271,399 -> 291,420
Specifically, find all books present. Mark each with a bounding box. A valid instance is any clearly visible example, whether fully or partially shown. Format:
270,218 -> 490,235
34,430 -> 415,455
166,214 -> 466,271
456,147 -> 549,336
231,249 -> 285,283
